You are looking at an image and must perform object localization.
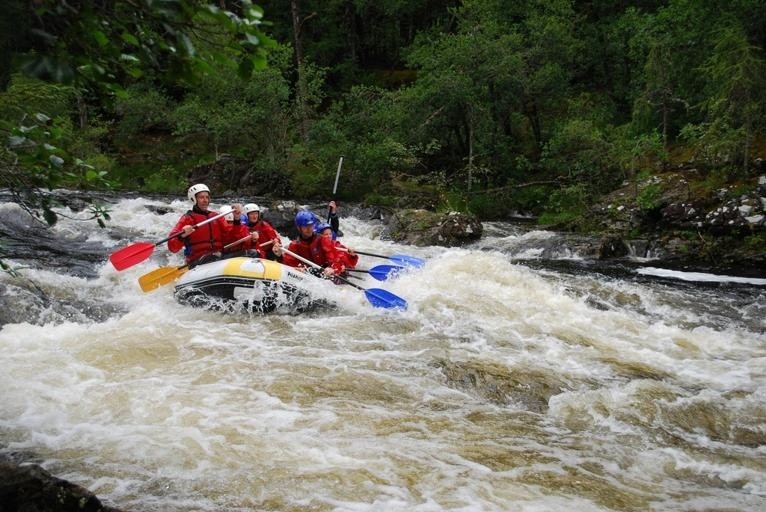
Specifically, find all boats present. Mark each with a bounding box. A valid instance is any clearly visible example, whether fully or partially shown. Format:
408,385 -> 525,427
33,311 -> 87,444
174,256 -> 339,315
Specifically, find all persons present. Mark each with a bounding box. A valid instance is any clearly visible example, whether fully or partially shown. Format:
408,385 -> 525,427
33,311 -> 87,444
168,184 -> 358,285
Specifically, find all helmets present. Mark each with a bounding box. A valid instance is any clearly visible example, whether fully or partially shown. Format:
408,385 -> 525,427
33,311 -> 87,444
187,184 -> 210,206
317,222 -> 332,235
244,203 -> 261,218
219,205 -> 249,226
294,210 -> 316,226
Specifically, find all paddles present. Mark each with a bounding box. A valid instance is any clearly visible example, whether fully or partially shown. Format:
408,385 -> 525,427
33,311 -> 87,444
340,265 -> 404,282
276,243 -> 408,311
138,231 -> 259,292
334,245 -> 425,269
108,206 -> 240,272
